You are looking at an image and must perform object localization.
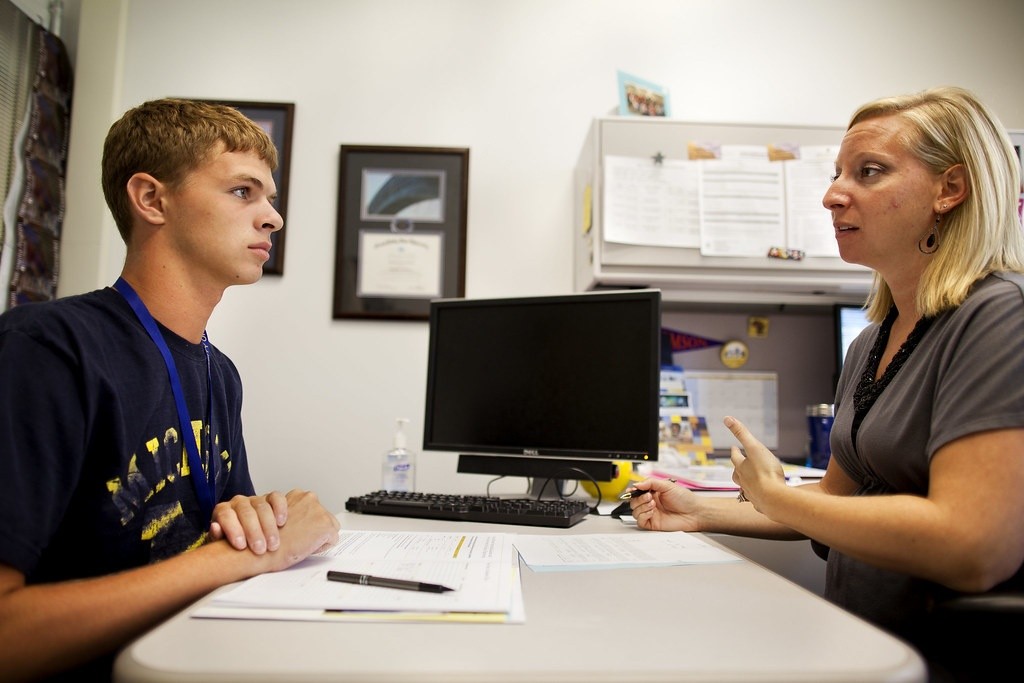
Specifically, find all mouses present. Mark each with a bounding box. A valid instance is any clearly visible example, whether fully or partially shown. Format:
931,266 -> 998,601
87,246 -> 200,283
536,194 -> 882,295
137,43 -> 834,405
610,501 -> 633,519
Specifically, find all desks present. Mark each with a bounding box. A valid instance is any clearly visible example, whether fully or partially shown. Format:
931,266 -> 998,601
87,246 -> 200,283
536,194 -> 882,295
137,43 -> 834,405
112,510 -> 932,683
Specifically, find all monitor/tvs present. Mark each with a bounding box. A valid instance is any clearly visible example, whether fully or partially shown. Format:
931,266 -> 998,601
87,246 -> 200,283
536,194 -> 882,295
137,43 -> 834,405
422,289 -> 662,463
833,302 -> 874,395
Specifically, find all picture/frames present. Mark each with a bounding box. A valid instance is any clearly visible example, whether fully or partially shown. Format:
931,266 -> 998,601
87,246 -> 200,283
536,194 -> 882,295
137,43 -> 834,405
165,96 -> 297,278
332,143 -> 471,321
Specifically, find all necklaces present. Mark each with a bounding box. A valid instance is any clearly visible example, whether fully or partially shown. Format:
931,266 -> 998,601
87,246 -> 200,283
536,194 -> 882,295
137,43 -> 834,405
849,294 -> 945,412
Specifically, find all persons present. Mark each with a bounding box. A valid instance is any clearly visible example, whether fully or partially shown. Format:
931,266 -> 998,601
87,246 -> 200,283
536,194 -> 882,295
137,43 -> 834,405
623,79 -> 1022,683
0,98 -> 337,681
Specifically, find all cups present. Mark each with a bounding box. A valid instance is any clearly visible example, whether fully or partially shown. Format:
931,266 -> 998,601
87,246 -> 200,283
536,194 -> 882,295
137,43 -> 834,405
801,402 -> 835,473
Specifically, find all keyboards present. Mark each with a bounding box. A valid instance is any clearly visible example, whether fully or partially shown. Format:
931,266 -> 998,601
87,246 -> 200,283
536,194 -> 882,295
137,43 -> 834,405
345,488 -> 590,529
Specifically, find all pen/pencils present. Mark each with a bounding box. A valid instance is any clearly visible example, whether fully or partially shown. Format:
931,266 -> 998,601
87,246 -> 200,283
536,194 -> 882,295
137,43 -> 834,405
324,570 -> 457,597
618,478 -> 681,501
